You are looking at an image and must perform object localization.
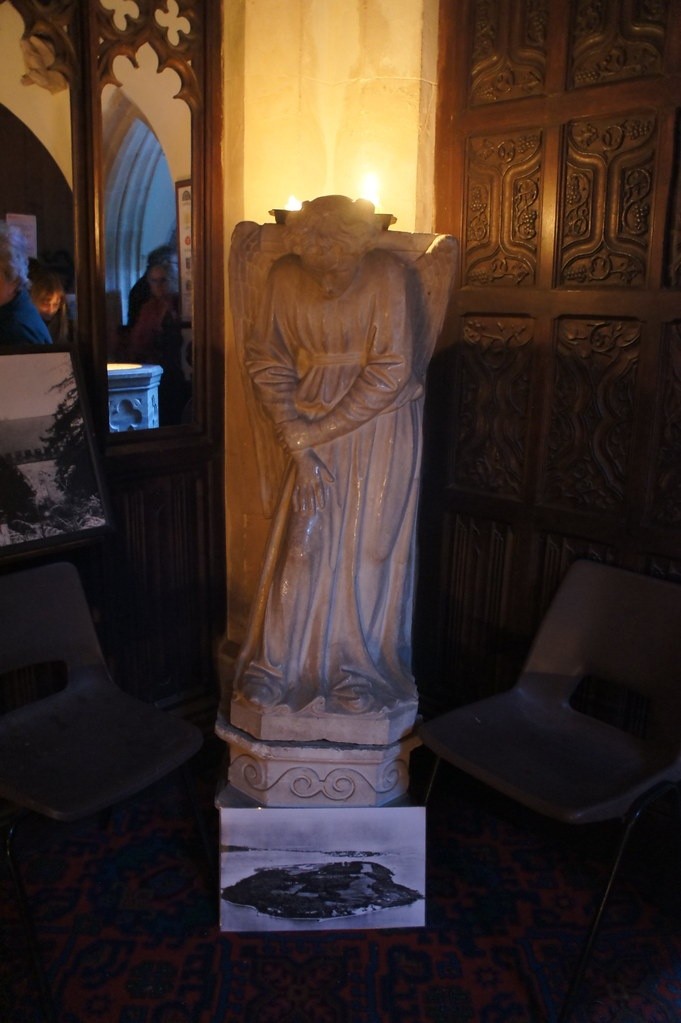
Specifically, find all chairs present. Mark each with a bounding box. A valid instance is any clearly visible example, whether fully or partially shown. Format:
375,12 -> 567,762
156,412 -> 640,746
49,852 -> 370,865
418,558 -> 681,1023
0,561 -> 217,1023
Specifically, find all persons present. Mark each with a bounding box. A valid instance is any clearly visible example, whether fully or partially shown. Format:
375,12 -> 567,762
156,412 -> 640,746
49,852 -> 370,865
0,224 -> 53,347
128,264 -> 192,425
127,246 -> 179,325
28,257 -> 71,346
234,195 -> 428,712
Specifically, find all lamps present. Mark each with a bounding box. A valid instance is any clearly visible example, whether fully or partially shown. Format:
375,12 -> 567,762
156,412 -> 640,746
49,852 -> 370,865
267,194 -> 398,231
